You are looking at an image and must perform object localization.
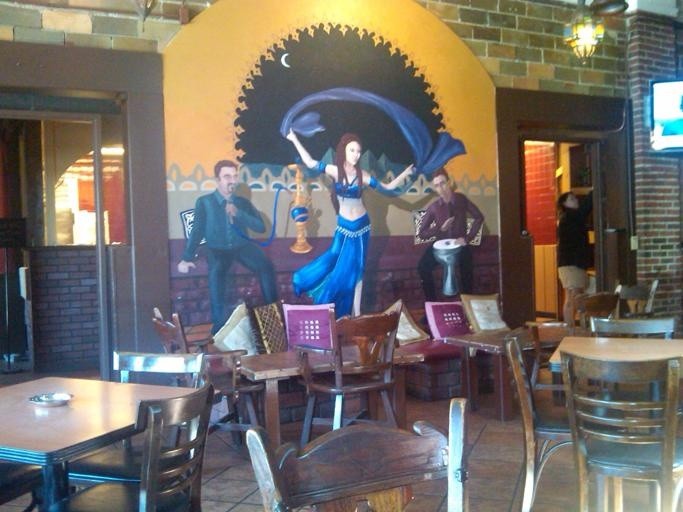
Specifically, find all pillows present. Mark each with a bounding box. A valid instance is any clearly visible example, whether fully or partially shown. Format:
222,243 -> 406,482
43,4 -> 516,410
461,294 -> 511,333
213,302 -> 260,358
384,299 -> 429,345
282,302 -> 335,354
425,300 -> 470,340
252,301 -> 288,354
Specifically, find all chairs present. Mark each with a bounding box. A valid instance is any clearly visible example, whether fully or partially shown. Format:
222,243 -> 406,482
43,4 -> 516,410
1,458 -> 45,512
525,288 -> 620,405
560,352 -> 683,512
246,397 -> 469,512
504,325 -> 623,512
302,300 -> 398,446
153,306 -> 265,446
562,1 -> 604,66
68,353 -> 204,494
591,316 -> 674,432
614,279 -> 658,320
65,382 -> 213,512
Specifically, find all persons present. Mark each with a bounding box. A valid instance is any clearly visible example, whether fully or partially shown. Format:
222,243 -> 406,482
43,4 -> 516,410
417,167 -> 485,324
177,160 -> 279,336
556,185 -> 594,327
286,128 -> 417,317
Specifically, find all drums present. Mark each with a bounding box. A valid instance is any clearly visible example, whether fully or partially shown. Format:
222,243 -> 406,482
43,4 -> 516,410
432,238 -> 466,298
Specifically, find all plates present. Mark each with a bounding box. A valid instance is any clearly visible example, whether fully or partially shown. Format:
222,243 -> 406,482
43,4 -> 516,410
28,391 -> 74,407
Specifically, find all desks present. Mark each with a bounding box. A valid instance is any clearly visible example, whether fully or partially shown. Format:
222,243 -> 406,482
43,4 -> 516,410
548,337 -> 683,512
445,320 -> 581,420
239,347 -> 425,450
0,377 -> 223,512
